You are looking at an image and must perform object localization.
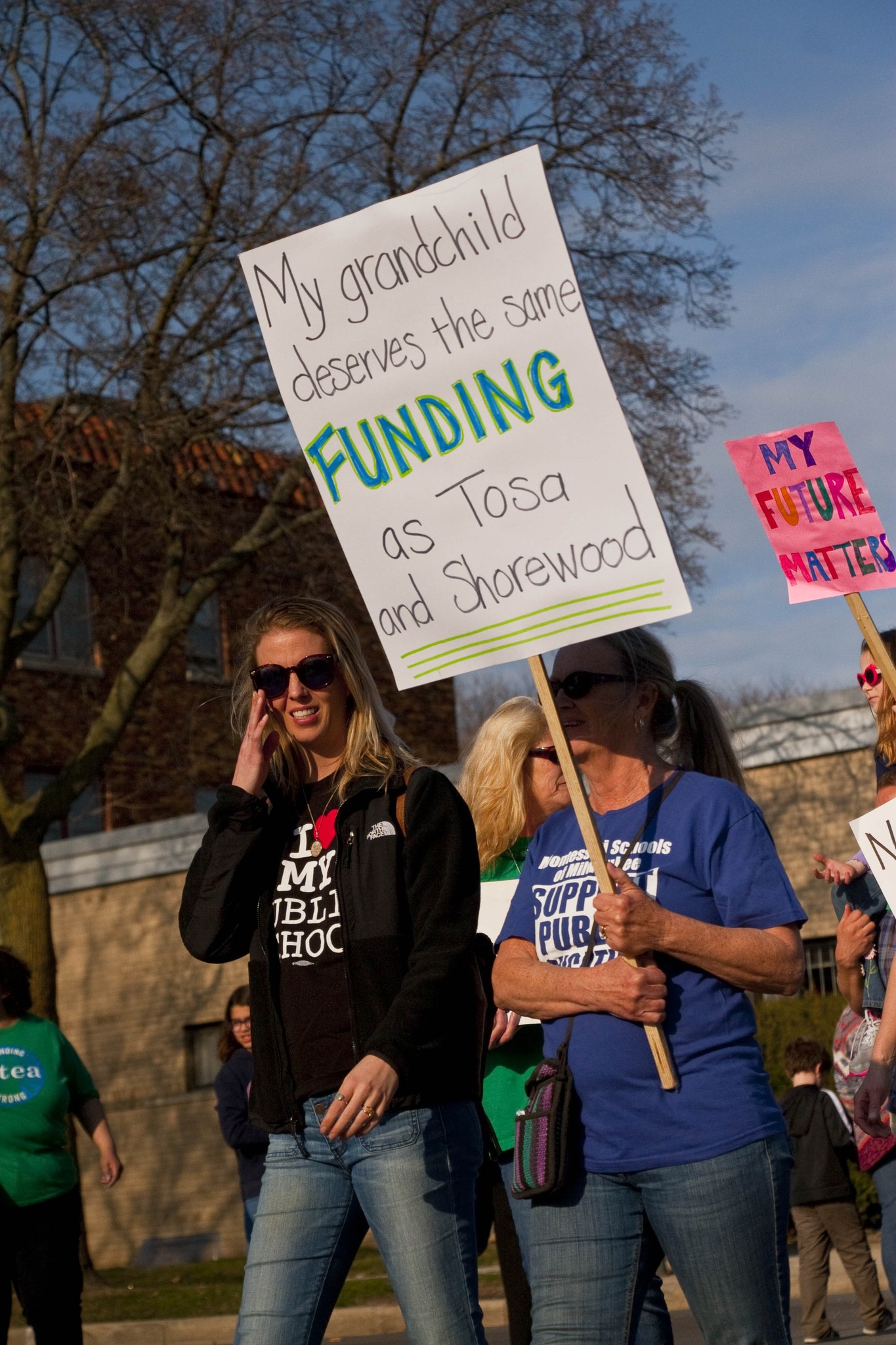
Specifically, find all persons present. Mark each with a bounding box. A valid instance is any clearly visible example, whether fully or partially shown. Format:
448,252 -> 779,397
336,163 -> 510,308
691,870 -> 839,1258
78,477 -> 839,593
812,628 -> 896,1301
178,595 -> 489,1345
456,626 -> 809,1345
0,944 -> 128,1345
777,1036 -> 896,1344
210,983 -> 269,1247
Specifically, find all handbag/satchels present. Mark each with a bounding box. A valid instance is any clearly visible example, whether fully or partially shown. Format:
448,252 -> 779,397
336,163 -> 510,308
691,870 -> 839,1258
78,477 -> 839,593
509,1060 -> 576,1201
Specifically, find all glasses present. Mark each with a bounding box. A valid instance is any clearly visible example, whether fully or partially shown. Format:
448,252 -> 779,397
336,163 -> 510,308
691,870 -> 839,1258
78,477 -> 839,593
537,670 -> 642,705
526,744 -> 559,766
231,1016 -> 251,1032
856,664 -> 882,690
249,643 -> 342,700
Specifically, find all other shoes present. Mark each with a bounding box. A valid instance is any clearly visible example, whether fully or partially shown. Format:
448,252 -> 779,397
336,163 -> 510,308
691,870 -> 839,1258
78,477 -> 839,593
803,1327 -> 840,1343
862,1309 -> 893,1335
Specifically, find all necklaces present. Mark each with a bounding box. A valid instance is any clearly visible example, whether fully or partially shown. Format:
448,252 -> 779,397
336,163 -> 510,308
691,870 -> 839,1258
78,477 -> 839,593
301,782 -> 340,858
508,847 -> 522,875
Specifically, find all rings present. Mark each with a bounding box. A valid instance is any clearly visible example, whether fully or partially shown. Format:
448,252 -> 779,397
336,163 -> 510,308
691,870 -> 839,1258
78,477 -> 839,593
362,1105 -> 376,1118
335,1093 -> 348,1105
601,926 -> 606,937
